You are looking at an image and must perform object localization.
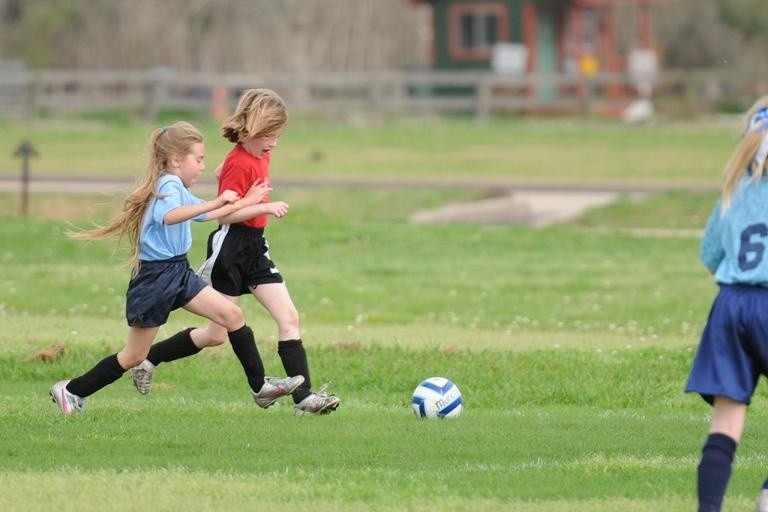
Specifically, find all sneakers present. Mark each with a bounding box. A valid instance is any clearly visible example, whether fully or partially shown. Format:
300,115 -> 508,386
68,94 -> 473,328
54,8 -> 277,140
49,378 -> 85,419
249,375 -> 305,409
132,359 -> 154,395
293,392 -> 340,418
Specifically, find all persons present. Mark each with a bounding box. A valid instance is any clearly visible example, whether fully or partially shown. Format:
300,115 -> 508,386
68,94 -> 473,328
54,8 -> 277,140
681,95 -> 768,512
132,89 -> 341,417
48,120 -> 304,414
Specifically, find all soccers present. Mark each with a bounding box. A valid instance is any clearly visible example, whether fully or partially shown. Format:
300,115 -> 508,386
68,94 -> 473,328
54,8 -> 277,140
413,377 -> 463,418
